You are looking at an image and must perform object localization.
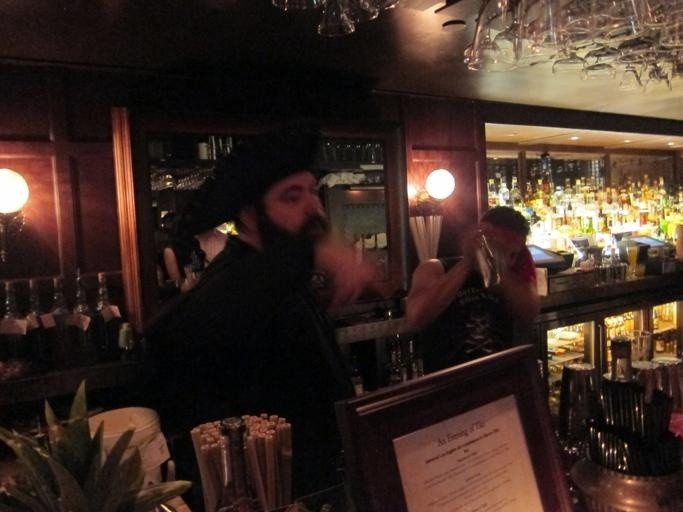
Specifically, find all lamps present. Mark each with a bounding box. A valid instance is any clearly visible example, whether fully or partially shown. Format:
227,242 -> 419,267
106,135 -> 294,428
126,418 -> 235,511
415,168 -> 455,217
0,168 -> 30,235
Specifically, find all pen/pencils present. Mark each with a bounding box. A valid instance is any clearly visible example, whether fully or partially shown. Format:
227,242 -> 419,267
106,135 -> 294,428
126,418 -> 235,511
191,413 -> 293,511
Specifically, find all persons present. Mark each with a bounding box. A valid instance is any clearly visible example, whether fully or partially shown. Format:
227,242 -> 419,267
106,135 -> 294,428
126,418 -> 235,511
397,206 -> 540,369
114,132 -> 394,498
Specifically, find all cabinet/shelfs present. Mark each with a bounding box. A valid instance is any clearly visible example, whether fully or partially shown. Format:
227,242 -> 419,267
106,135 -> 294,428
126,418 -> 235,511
485,147 -> 683,417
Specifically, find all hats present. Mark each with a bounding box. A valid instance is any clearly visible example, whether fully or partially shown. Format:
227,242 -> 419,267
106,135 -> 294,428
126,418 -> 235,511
194,134 -> 321,230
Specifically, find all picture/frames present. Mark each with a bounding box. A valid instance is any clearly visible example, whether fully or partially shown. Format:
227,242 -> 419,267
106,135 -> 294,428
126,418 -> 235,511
340,347 -> 577,512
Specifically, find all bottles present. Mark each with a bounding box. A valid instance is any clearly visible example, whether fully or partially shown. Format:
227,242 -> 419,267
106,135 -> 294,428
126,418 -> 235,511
0,271 -> 124,376
487,176 -> 683,241
